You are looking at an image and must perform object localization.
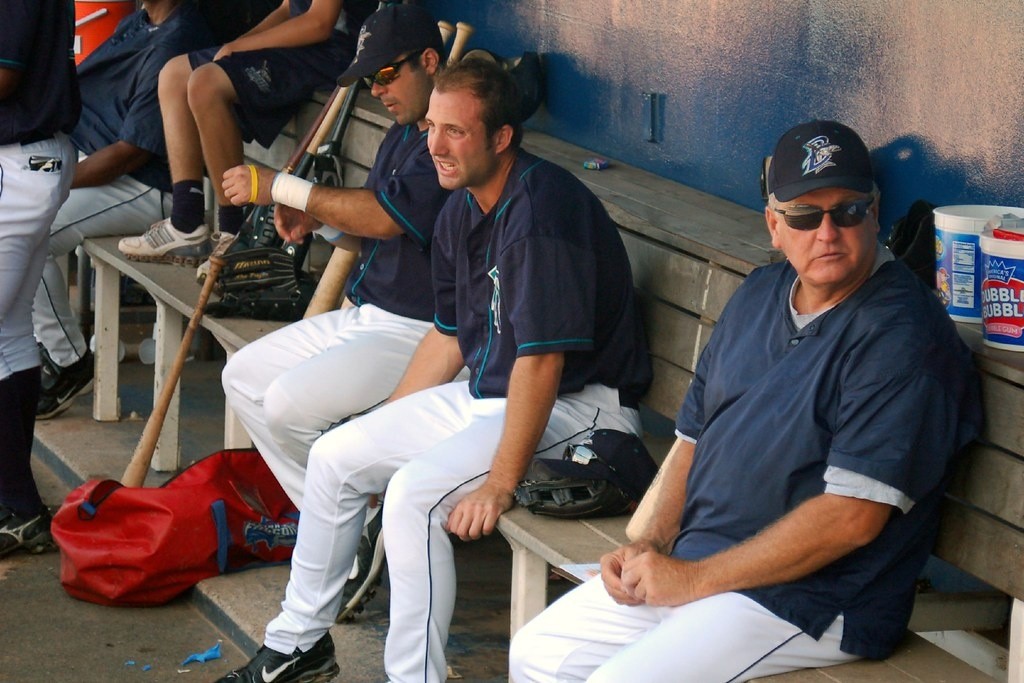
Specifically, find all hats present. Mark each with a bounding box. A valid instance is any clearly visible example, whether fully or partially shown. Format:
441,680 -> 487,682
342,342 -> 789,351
768,120 -> 873,202
336,5 -> 439,87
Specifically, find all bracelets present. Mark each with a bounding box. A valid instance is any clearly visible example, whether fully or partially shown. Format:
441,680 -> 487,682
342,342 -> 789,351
271,171 -> 313,212
312,225 -> 344,243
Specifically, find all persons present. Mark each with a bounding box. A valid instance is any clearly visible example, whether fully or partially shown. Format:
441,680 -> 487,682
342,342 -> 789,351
221,3 -> 454,625
115,0 -> 380,285
28,0 -> 215,420
0,0 -> 84,557
217,58 -> 650,683
509,118 -> 969,683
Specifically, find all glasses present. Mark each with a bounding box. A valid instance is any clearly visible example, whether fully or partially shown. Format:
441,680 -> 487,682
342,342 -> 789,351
776,195 -> 875,231
363,51 -> 423,88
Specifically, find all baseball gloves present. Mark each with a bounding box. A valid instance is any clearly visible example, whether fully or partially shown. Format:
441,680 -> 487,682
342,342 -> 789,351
884,200 -> 937,289
512,427 -> 657,520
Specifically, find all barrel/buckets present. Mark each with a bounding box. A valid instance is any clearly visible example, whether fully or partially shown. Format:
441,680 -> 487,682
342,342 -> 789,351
932,204 -> 1024,325
977,228 -> 1024,354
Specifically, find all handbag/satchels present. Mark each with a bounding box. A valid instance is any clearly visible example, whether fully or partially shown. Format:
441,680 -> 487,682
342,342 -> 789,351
50,448 -> 300,606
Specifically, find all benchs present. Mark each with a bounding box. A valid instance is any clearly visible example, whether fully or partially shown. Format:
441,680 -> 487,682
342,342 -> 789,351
78,73 -> 1024,681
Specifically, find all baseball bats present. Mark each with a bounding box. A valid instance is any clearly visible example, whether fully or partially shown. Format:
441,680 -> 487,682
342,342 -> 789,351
301,21 -> 455,321
120,256 -> 226,488
225,57 -> 363,268
339,22 -> 475,311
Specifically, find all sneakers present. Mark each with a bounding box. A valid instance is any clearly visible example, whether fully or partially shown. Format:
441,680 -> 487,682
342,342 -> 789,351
215,632 -> 339,683
335,500 -> 384,622
118,216 -> 212,264
197,231 -> 236,284
35,349 -> 94,419
0,506 -> 51,556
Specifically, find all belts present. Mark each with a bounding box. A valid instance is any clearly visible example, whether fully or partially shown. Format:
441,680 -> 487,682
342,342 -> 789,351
20,132 -> 54,146
